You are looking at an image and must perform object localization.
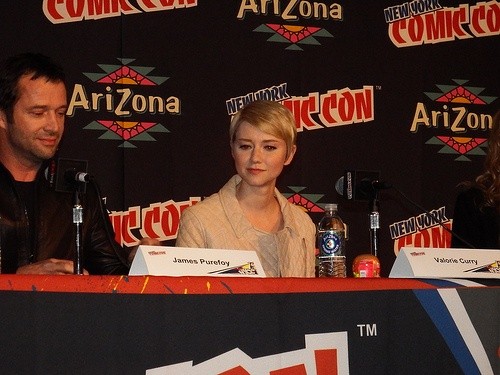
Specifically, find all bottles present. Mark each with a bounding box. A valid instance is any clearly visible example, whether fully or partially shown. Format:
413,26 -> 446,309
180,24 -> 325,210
317,204 -> 347,277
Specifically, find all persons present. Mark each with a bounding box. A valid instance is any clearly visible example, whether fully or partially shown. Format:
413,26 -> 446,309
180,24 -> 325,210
0,52 -> 161,276
176,99 -> 316,278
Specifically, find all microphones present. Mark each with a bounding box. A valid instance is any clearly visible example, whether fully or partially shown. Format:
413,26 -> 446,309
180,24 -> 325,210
44,158 -> 94,193
334,168 -> 392,200
